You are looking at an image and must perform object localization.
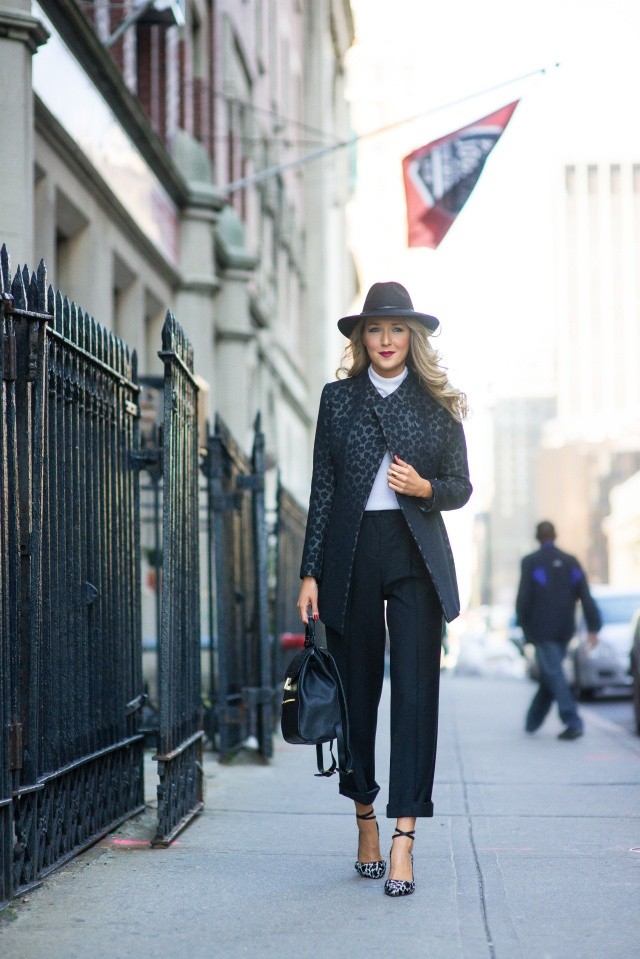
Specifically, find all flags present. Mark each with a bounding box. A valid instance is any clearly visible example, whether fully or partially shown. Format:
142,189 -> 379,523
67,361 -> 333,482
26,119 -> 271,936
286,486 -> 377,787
399,93 -> 524,249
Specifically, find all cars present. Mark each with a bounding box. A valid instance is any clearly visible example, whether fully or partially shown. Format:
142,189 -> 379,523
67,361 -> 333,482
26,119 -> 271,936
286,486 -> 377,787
561,589 -> 640,701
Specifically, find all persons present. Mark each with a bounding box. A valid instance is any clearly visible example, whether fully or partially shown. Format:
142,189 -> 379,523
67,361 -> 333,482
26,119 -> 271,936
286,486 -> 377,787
294,281 -> 471,896
515,520 -> 603,741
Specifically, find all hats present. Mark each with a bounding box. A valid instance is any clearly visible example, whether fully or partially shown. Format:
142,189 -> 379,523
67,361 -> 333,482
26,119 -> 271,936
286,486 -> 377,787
337,282 -> 440,339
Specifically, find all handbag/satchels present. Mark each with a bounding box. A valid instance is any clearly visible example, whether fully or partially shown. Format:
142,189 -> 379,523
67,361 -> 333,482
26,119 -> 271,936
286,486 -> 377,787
280,617 -> 352,777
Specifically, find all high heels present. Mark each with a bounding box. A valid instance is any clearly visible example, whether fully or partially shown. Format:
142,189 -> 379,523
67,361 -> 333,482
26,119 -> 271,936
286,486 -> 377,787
384,828 -> 415,899
355,810 -> 386,879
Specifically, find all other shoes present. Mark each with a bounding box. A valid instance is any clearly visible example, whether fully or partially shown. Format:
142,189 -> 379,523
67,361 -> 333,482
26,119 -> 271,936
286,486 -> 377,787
559,729 -> 583,741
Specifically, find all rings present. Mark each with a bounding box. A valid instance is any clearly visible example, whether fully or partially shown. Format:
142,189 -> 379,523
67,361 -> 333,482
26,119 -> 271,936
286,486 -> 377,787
391,471 -> 399,478
404,463 -> 409,467
